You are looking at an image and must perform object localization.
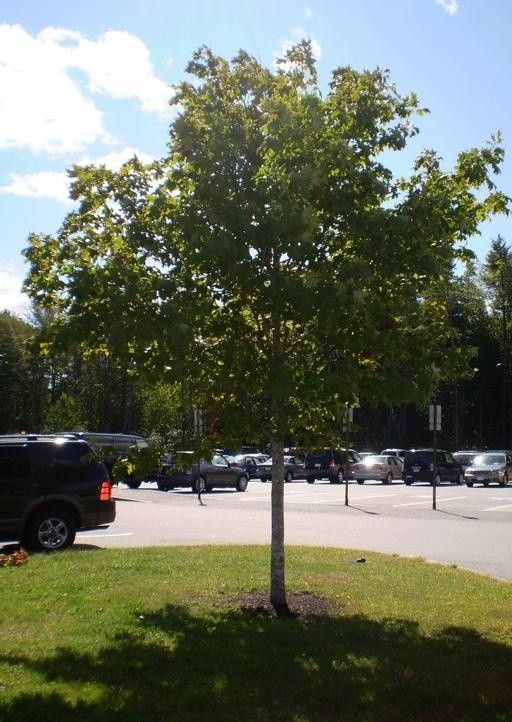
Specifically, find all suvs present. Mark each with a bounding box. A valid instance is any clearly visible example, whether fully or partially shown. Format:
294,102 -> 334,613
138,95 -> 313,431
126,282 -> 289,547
380,448 -> 408,475
57,432 -> 156,489
402,448 -> 465,487
306,447 -> 363,484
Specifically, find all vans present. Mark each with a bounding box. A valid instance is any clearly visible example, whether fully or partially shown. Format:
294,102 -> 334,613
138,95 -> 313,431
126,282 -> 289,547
0,434 -> 116,552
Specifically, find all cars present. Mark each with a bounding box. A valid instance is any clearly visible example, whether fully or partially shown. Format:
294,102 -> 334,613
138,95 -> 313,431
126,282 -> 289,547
351,455 -> 404,484
156,450 -> 248,493
356,452 -> 378,461
453,451 -> 482,473
464,454 -> 512,488
208,449 -> 304,483
